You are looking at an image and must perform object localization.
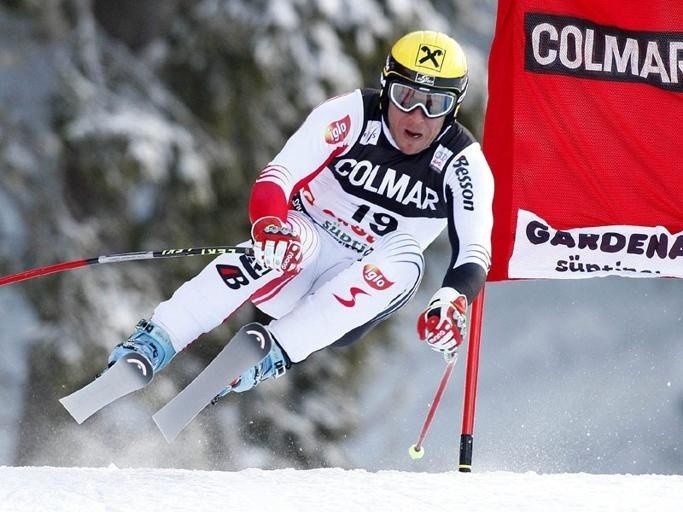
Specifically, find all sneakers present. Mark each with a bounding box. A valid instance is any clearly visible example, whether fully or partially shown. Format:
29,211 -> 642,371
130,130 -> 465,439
106,318 -> 175,377
215,323 -> 289,399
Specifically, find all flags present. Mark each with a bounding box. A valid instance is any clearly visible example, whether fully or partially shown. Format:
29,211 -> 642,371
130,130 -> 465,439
482,1 -> 683,281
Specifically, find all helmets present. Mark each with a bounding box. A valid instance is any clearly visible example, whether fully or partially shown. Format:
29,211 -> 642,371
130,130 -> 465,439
380,30 -> 470,120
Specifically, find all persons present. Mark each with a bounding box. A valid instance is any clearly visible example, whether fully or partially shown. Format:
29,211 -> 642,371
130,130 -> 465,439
100,29 -> 494,411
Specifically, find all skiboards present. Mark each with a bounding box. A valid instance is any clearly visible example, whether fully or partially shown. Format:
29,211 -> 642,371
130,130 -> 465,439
58,321 -> 273,444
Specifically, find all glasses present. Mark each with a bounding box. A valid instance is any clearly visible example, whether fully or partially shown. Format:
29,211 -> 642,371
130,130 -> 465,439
384,79 -> 459,120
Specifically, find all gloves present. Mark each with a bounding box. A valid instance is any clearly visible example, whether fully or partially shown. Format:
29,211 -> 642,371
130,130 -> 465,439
416,293 -> 470,355
249,214 -> 304,276
